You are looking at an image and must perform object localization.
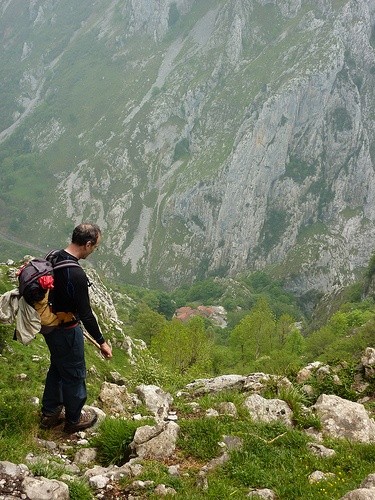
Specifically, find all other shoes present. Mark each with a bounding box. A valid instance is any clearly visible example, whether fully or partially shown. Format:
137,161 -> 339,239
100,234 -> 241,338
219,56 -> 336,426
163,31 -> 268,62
40,411 -> 65,428
63,409 -> 97,433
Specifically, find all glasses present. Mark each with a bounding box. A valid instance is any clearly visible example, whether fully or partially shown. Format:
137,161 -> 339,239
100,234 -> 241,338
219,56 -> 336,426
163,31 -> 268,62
91,242 -> 100,253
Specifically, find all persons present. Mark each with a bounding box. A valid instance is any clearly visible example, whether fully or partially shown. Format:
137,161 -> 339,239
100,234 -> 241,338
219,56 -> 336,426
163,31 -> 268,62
36,223 -> 113,433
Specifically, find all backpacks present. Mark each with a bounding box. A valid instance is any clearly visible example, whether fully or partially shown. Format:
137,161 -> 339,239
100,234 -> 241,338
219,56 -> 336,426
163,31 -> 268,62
19,250 -> 82,326
